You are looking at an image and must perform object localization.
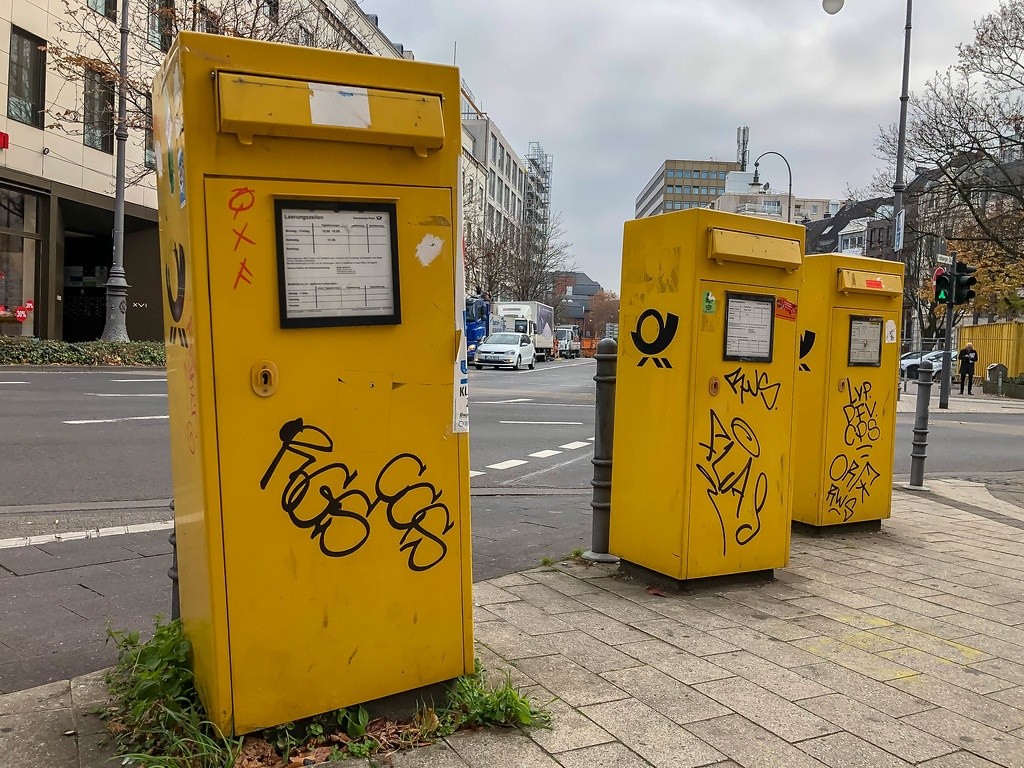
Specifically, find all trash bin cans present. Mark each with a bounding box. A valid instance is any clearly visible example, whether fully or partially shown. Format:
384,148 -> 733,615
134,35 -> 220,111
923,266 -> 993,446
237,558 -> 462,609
986,363 -> 1009,382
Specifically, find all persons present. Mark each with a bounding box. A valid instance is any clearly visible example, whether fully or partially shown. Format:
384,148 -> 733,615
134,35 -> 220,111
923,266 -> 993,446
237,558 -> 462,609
958,342 -> 978,397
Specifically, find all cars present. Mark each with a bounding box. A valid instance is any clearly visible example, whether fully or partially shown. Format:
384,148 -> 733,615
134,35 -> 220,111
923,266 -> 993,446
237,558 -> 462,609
473,332 -> 536,371
900,350 -> 958,381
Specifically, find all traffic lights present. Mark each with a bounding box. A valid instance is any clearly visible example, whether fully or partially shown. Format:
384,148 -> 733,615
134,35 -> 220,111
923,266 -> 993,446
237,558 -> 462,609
956,262 -> 977,274
955,275 -> 977,304
935,275 -> 950,301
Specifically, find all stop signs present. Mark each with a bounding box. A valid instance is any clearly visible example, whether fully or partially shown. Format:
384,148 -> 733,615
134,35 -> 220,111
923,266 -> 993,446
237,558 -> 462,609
932,267 -> 944,295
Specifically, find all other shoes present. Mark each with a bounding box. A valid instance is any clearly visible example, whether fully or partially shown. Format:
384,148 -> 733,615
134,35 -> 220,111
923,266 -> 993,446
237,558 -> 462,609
958,391 -> 963,395
968,390 -> 974,395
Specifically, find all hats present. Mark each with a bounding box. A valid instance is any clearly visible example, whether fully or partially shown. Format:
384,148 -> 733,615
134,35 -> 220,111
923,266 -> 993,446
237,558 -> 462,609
519,326 -> 523,331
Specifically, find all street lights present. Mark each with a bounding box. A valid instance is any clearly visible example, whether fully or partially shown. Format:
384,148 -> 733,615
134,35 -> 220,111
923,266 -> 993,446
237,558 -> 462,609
748,152 -> 792,223
822,0 -> 912,261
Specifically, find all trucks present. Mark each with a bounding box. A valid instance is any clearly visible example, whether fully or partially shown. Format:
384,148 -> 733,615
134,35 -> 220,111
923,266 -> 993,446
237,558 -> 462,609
466,295 -> 516,366
552,325 -> 580,359
493,302 -> 554,362
